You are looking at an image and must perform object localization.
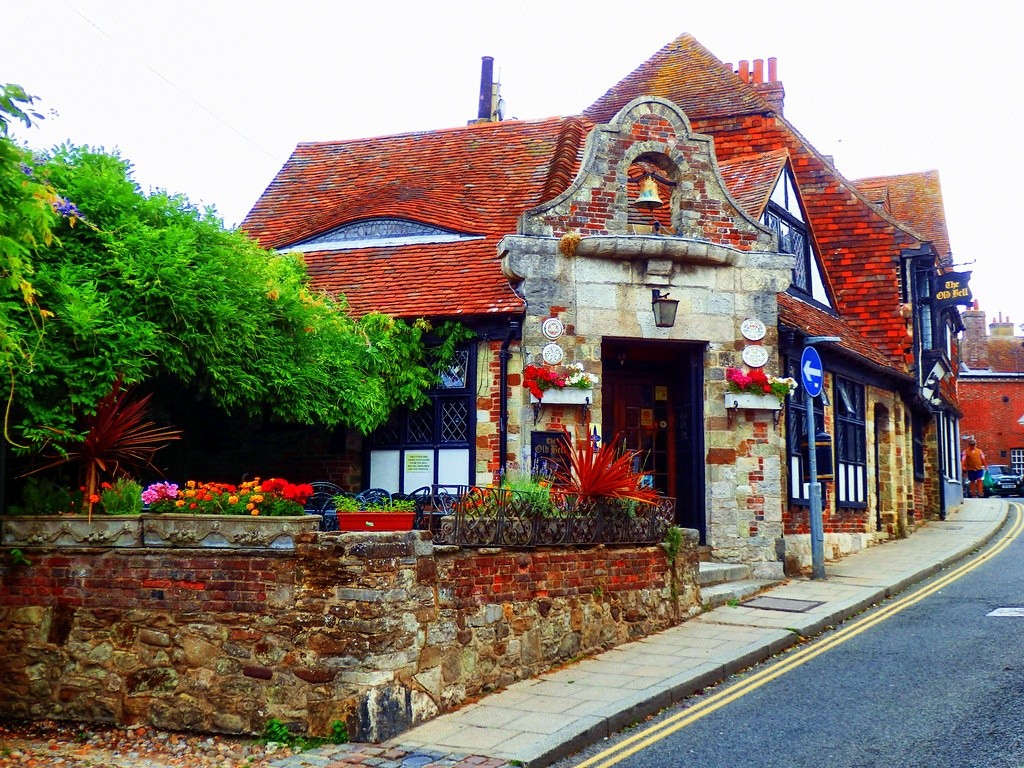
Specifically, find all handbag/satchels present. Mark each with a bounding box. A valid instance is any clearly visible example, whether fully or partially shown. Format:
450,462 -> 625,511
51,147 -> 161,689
983,471 -> 994,488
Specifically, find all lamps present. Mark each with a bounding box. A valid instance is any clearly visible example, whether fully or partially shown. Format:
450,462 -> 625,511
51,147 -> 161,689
652,289 -> 679,328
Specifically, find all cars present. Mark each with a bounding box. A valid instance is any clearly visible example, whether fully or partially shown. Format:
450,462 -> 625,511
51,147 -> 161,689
962,463 -> 1024,499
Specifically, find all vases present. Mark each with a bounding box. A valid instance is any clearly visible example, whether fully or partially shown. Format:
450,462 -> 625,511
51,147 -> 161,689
0,513 -> 323,550
529,386 -> 594,405
442,515 -> 668,547
724,392 -> 783,410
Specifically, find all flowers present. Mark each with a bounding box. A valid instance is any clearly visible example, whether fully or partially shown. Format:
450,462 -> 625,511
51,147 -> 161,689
520,361 -> 600,399
439,419 -> 671,516
70,477 -> 315,516
726,366 -> 798,404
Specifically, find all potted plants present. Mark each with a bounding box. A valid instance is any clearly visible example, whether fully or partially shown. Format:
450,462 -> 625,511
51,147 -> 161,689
335,494 -> 416,532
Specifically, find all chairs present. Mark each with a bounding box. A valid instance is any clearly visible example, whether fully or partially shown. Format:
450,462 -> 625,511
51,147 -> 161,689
301,479 -> 431,532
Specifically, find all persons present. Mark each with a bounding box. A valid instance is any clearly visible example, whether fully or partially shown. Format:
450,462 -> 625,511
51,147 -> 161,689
962,439 -> 989,499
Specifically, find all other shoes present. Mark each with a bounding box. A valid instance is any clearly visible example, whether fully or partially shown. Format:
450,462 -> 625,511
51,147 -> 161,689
978,494 -> 983,497
972,494 -> 978,498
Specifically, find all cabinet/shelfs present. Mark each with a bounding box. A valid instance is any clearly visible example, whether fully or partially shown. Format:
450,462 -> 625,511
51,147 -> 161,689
615,377 -> 676,510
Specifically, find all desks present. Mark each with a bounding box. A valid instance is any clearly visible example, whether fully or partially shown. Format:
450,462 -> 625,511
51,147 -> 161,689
303,510 -> 339,531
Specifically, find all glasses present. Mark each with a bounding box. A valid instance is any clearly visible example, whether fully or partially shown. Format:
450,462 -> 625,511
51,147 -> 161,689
968,444 -> 975,446
